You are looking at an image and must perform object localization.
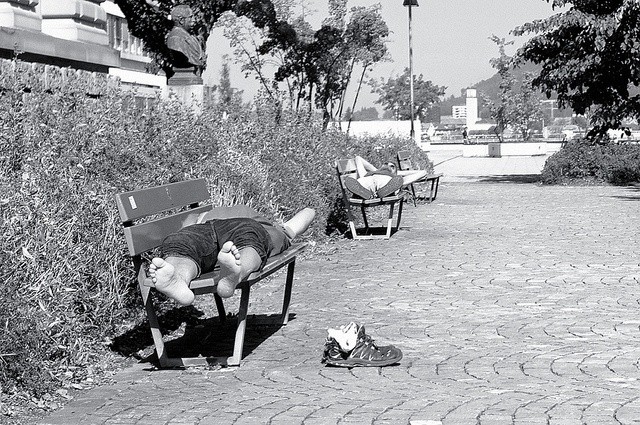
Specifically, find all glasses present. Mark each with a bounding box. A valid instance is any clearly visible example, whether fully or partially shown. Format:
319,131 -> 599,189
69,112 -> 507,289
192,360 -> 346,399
385,162 -> 394,169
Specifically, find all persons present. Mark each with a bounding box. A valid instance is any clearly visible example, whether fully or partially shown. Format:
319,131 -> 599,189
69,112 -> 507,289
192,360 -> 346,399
462,127 -> 472,144
165,5 -> 207,70
344,156 -> 428,200
147,206 -> 315,306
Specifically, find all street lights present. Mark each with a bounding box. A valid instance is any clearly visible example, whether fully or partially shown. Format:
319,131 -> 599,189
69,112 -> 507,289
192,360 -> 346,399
403,0 -> 419,137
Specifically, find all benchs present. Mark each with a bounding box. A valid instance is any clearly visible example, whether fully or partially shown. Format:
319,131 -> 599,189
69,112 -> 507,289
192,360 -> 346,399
397,150 -> 443,203
336,159 -> 408,240
114,177 -> 308,368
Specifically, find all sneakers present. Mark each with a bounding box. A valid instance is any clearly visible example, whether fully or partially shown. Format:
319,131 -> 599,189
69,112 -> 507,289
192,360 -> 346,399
322,322 -> 403,367
373,175 -> 404,199
345,176 -> 374,199
